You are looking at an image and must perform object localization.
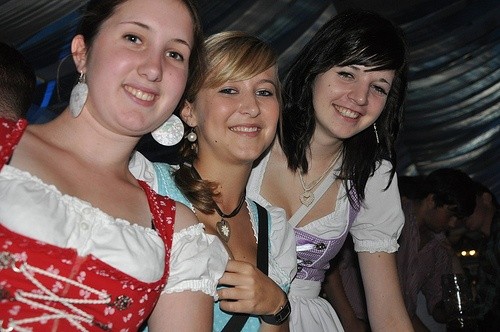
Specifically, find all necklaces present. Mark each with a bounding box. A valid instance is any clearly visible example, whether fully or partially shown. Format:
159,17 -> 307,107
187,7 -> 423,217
190,164 -> 246,242
297,143 -> 344,208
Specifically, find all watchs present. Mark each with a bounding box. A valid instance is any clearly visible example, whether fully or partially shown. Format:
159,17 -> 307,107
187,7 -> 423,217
258,289 -> 292,325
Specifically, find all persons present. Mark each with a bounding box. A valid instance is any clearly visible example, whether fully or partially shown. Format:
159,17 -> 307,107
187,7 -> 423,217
0,0 -> 230,332
461,180 -> 500,267
242,7 -> 415,332
127,24 -> 298,332
0,42 -> 36,123
432,223 -> 500,317
318,166 -> 477,332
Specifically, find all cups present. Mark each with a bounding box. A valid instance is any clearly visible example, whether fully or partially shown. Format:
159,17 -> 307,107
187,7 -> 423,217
441,273 -> 476,332
